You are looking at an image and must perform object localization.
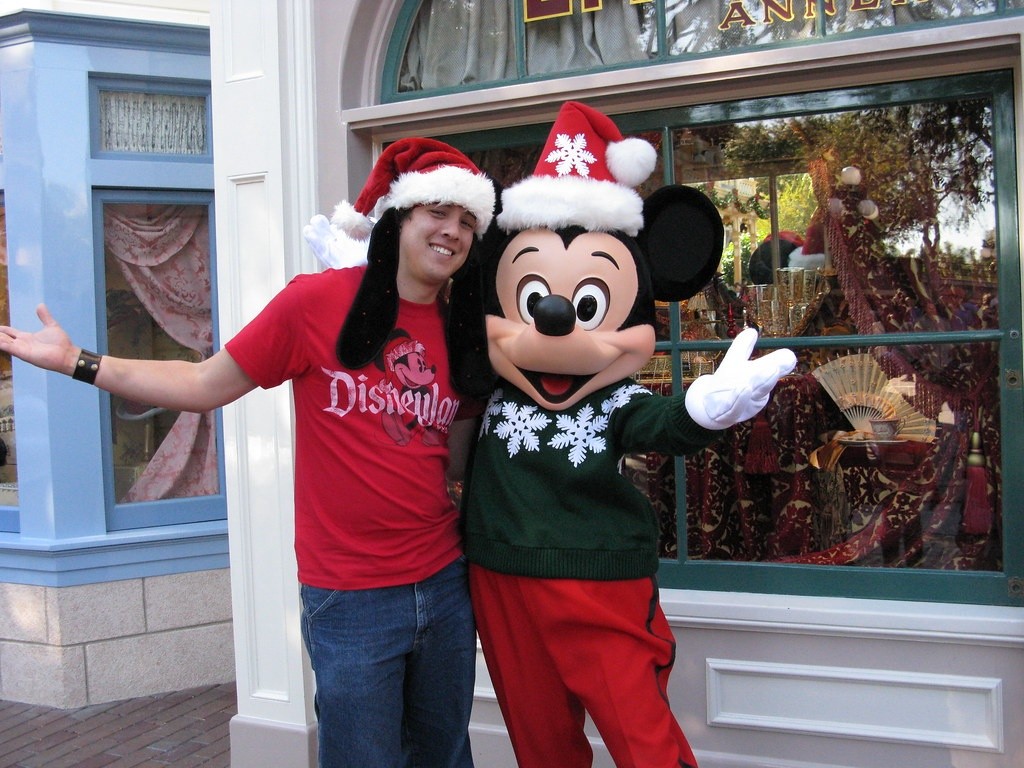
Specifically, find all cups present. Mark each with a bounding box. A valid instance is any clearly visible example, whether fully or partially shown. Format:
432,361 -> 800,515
745,266 -> 815,339
869,418 -> 905,440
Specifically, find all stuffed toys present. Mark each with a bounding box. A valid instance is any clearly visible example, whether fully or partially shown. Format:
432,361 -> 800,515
303,100 -> 799,768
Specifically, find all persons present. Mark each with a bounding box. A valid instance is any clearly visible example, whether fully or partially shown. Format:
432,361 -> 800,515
0,136 -> 496,768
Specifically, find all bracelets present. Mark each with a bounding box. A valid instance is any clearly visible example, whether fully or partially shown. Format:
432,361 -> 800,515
72,349 -> 103,385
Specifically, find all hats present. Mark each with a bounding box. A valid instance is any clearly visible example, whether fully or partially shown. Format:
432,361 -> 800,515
333,136 -> 495,238
